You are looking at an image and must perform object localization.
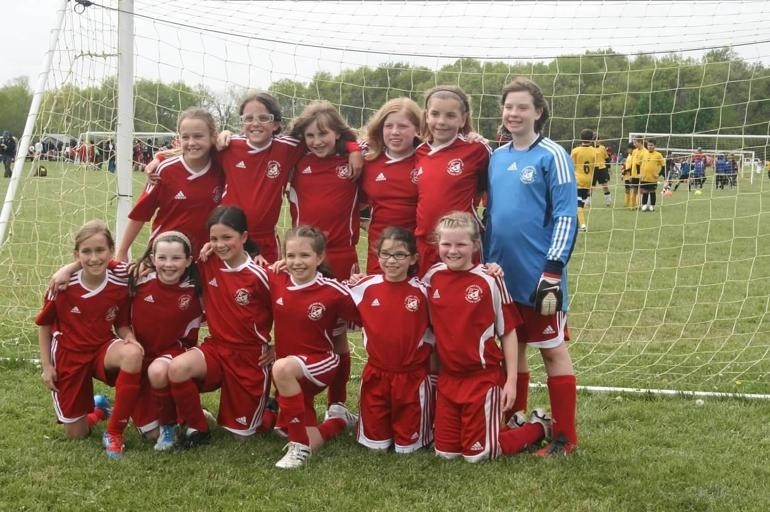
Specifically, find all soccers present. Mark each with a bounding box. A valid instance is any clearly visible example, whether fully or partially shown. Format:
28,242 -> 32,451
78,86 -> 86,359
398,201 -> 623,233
663,191 -> 673,197
694,189 -> 702,196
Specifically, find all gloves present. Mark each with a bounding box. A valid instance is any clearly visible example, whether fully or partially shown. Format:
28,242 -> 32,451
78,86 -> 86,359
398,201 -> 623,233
529,259 -> 566,318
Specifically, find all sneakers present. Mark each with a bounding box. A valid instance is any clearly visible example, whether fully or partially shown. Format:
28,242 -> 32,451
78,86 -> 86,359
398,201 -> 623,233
153,425 -> 181,452
507,410 -> 529,426
328,405 -> 359,429
101,430 -> 124,462
95,393 -> 113,417
275,441 -> 313,471
535,444 -> 579,458
580,225 -> 585,231
200,406 -> 219,428
530,408 -> 553,441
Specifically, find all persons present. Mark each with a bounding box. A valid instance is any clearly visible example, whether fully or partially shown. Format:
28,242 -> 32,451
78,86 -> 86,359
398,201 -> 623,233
410,85 -> 493,277
419,213 -> 551,463
592,135 -> 611,207
345,224 -> 435,452
257,225 -> 365,468
569,130 -> 597,232
26,138 -> 157,172
631,136 -> 646,211
165,206 -> 275,449
356,96 -> 483,277
661,146 -> 712,194
478,78 -> 578,460
625,144 -> 635,205
55,231 -> 218,439
639,139 -> 666,212
605,146 -> 623,164
0,131 -> 16,177
217,98 -> 361,285
109,107 -> 222,264
140,89 -> 363,265
714,153 -> 739,192
34,219 -> 143,459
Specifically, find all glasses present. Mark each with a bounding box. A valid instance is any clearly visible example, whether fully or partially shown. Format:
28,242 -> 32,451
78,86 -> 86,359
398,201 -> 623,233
377,249 -> 415,261
239,113 -> 276,126
